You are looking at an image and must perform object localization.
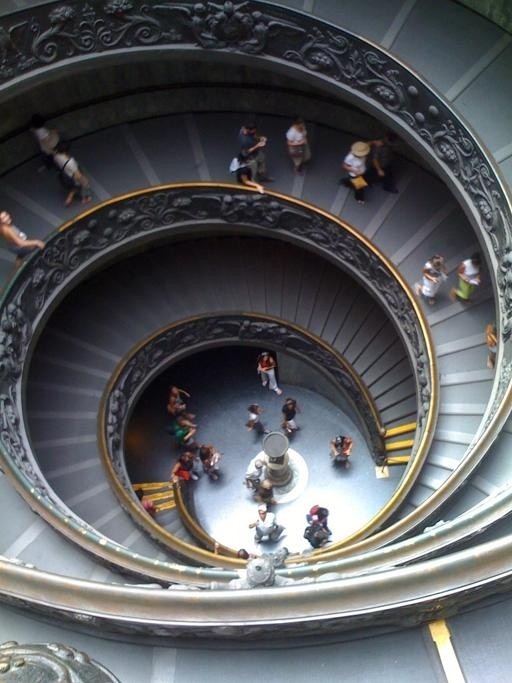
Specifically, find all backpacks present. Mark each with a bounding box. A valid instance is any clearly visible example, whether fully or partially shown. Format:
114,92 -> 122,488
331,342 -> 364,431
38,159 -> 73,205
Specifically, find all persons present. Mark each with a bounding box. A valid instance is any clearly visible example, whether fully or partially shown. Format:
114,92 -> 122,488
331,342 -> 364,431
28,112 -> 60,154
52,142 -> 94,204
230,152 -> 265,195
448,253 -> 484,304
238,122 -> 274,182
416,254 -> 448,309
486,321 -> 496,368
165,346 -> 351,546
343,139 -> 384,205
284,117 -> 314,178
373,133 -> 398,183
0,211 -> 48,264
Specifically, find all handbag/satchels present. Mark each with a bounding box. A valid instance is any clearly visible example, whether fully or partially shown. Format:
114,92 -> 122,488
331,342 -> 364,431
486,323 -> 496,347
164,404 -> 338,549
288,144 -> 306,159
349,175 -> 368,190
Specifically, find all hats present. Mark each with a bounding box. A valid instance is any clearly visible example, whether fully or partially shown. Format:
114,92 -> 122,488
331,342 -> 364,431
35,125 -> 61,154
350,140 -> 371,157
